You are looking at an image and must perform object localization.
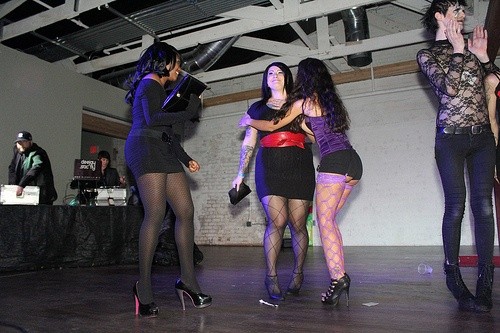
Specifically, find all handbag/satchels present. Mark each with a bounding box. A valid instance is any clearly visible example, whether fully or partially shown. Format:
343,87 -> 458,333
161,74 -> 207,114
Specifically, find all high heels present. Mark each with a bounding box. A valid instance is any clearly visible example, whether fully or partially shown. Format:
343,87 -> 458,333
175,279 -> 212,310
321,272 -> 351,304
131,280 -> 159,319
285,271 -> 304,295
321,276 -> 349,307
443,261 -> 476,310
473,263 -> 495,312
264,274 -> 284,301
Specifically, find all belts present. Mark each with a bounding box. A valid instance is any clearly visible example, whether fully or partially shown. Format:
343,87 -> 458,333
436,124 -> 492,135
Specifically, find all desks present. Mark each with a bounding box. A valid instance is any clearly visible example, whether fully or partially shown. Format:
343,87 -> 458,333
0,204 -> 204,275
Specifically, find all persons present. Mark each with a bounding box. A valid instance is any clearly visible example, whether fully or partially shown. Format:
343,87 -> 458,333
97,149 -> 121,187
414,0 -> 496,313
233,60 -> 319,301
483,41 -> 500,251
124,40 -> 213,319
238,56 -> 364,308
8,130 -> 57,204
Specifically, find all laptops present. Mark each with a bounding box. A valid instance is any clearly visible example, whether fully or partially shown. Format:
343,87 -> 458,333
74,159 -> 102,177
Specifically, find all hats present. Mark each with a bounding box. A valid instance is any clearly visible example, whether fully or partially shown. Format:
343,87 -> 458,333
14,131 -> 32,142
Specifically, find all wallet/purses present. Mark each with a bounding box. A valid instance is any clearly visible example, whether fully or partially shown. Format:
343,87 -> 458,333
228,181 -> 252,206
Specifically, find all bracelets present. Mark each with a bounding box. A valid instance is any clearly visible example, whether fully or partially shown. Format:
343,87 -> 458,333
238,172 -> 246,176
